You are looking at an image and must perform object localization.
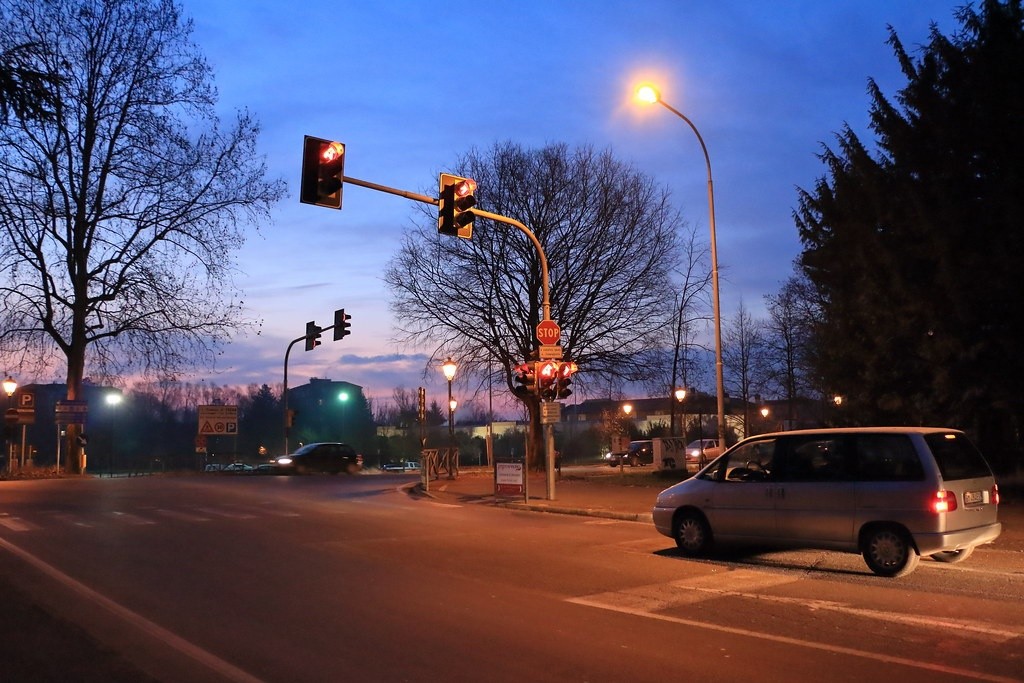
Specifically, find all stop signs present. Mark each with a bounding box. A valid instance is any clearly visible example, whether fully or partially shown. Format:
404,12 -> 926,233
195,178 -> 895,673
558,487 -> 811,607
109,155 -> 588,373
536,320 -> 561,345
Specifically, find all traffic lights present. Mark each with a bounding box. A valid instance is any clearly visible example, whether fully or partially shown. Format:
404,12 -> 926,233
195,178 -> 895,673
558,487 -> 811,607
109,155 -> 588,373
300,133 -> 346,211
305,321 -> 322,351
556,362 -> 572,399
437,171 -> 477,239
538,361 -> 556,400
333,308 -> 351,342
515,362 -> 538,396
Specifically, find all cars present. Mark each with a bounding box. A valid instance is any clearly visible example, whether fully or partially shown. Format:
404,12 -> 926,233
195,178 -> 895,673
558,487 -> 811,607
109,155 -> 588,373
684,438 -> 726,464
381,461 -> 422,471
603,439 -> 654,467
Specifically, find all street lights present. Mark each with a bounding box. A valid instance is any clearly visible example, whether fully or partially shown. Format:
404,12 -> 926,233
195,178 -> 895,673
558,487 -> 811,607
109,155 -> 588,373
105,393 -> 121,477
2,375 -> 18,477
675,389 -> 686,436
631,80 -> 726,460
762,408 -> 770,434
622,405 -> 633,452
442,356 -> 458,480
449,395 -> 458,453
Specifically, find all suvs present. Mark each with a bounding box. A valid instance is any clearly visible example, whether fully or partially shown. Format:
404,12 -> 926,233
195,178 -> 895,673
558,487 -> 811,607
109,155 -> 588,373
269,440 -> 359,476
649,423 -> 1003,578
